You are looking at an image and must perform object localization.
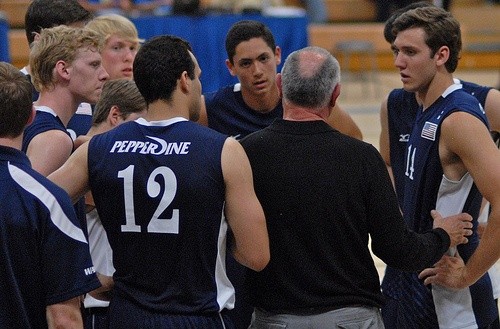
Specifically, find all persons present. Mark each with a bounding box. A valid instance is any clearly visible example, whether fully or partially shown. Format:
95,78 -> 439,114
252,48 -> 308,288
0,61 -> 102,329
0,0 -> 500,329
47,36 -> 270,329
224,46 -> 472,329
379,7 -> 500,329
